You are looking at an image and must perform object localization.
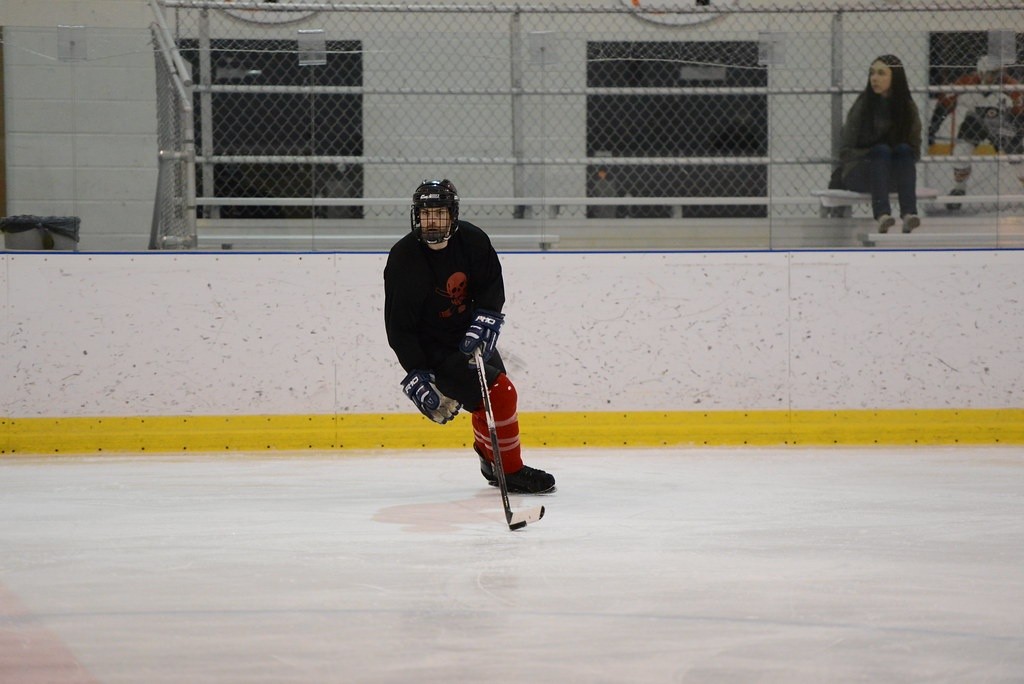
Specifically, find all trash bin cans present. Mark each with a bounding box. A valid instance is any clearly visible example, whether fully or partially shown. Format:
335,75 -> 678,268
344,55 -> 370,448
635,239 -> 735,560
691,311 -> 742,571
1,214 -> 82,251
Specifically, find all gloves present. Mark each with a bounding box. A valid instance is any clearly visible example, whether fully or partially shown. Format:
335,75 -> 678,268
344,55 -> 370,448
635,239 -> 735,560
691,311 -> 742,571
399,370 -> 461,424
459,313 -> 505,368
928,126 -> 937,145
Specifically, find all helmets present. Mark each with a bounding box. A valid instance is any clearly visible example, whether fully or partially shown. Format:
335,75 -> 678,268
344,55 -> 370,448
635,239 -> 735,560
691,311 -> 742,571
412,178 -> 458,244
978,56 -> 1002,87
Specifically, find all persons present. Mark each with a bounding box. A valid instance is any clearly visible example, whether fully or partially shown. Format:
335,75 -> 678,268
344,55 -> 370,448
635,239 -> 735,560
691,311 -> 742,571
383,178 -> 556,497
928,55 -> 1024,210
836,54 -> 923,234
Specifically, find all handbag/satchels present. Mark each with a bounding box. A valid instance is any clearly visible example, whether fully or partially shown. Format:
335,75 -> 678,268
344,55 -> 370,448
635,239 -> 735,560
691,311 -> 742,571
828,167 -> 846,190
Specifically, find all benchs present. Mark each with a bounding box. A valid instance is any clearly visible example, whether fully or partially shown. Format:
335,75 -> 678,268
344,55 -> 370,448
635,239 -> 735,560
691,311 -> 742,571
161,234 -> 559,252
856,231 -> 1024,248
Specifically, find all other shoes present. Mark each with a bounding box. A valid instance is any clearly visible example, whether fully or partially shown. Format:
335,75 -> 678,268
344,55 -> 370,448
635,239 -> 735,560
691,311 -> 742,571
945,189 -> 965,211
902,214 -> 919,233
878,216 -> 894,233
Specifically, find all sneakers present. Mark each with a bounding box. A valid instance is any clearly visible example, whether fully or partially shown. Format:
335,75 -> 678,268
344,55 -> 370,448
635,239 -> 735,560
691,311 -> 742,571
479,462 -> 499,486
496,464 -> 557,496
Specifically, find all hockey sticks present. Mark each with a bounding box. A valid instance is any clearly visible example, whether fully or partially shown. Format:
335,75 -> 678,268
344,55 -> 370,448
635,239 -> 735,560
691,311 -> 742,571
472,348 -> 546,532
